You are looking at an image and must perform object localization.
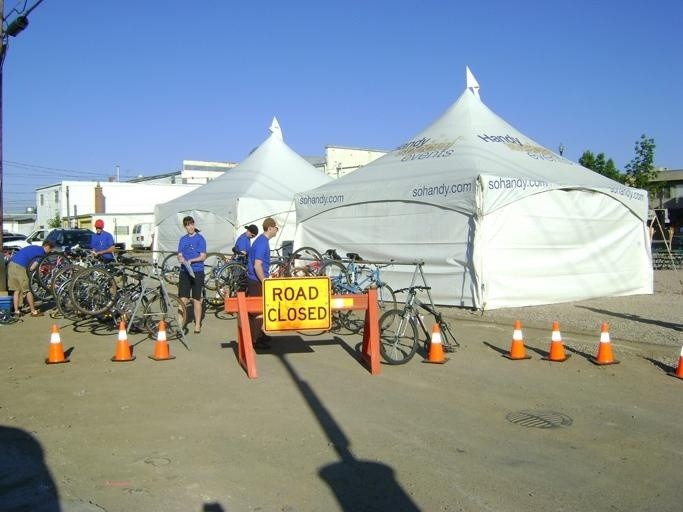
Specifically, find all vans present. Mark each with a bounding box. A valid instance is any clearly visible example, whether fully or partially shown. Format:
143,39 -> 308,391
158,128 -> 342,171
130,223 -> 154,249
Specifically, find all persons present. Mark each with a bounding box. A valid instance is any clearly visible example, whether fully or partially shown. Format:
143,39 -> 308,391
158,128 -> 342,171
5,240 -> 54,317
175,216 -> 207,335
87,219 -> 115,263
244,217 -> 279,350
230,224 -> 258,294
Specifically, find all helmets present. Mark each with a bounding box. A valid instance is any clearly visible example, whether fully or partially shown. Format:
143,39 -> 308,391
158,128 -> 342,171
94,219 -> 104,228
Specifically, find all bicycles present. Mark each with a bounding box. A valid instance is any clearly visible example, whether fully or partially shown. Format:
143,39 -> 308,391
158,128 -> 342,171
161,242 -> 396,333
0,238 -> 187,338
377,286 -> 459,365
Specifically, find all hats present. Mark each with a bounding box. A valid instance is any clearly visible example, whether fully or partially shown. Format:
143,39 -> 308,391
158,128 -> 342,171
244,224 -> 258,235
263,217 -> 276,228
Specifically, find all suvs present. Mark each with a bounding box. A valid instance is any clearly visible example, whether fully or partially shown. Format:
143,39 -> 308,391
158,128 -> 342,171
42,228 -> 125,258
1,225 -> 80,258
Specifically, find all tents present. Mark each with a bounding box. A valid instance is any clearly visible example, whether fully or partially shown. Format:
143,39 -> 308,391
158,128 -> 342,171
150,132 -> 337,278
291,86 -> 657,312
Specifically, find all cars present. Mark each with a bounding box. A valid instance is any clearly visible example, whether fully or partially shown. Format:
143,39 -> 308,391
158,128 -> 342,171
1,230 -> 39,243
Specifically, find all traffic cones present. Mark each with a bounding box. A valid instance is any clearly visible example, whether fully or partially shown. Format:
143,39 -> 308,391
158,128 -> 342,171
502,318 -> 532,360
44,321 -> 70,365
540,319 -> 571,362
588,321 -> 620,366
666,341 -> 682,380
147,318 -> 176,361
422,322 -> 450,364
110,318 -> 136,362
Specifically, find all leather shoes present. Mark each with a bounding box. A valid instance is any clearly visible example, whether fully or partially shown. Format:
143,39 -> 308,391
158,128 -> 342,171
13,312 -> 24,317
30,311 -> 43,316
